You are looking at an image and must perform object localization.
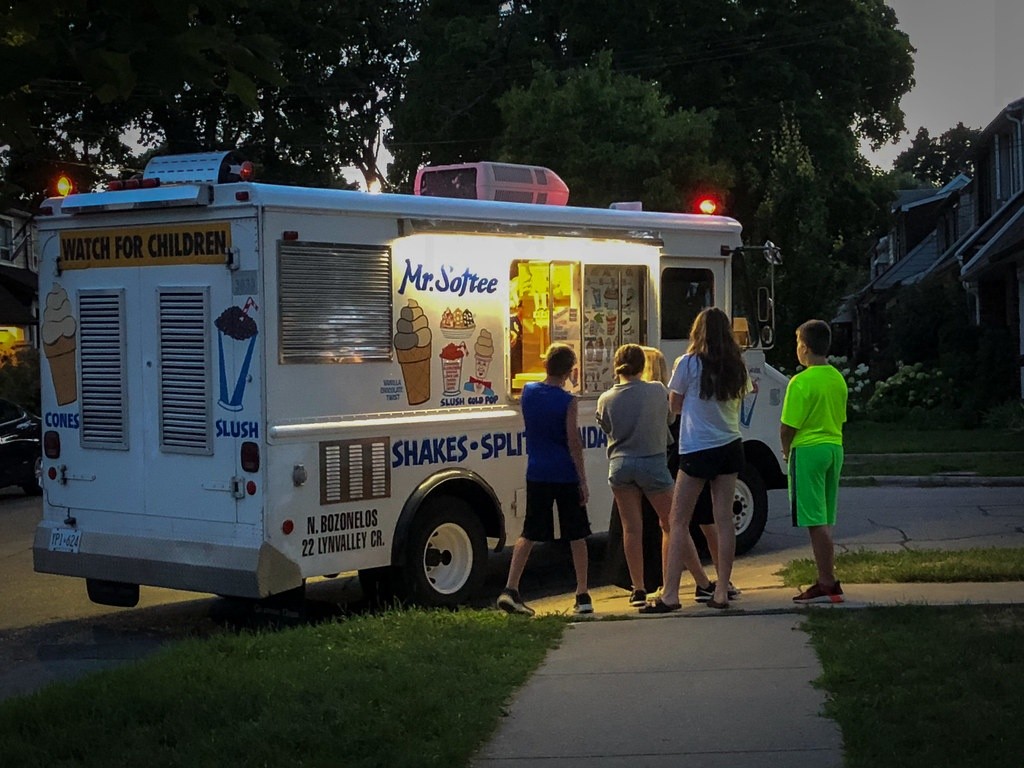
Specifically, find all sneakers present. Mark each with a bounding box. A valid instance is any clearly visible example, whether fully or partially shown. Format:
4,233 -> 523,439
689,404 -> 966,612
572,593 -> 594,613
629,588 -> 647,605
695,582 -> 736,603
792,580 -> 845,603
498,589 -> 534,616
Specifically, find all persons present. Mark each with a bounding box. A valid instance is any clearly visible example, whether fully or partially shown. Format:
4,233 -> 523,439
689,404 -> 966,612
595,308 -> 750,613
496,343 -> 594,615
780,320 -> 848,603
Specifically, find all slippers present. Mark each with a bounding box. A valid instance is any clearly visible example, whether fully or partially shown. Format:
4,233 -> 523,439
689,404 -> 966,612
639,597 -> 683,613
707,595 -> 729,608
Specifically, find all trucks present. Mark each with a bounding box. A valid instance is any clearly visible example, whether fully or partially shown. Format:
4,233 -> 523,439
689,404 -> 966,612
29,151 -> 790,611
